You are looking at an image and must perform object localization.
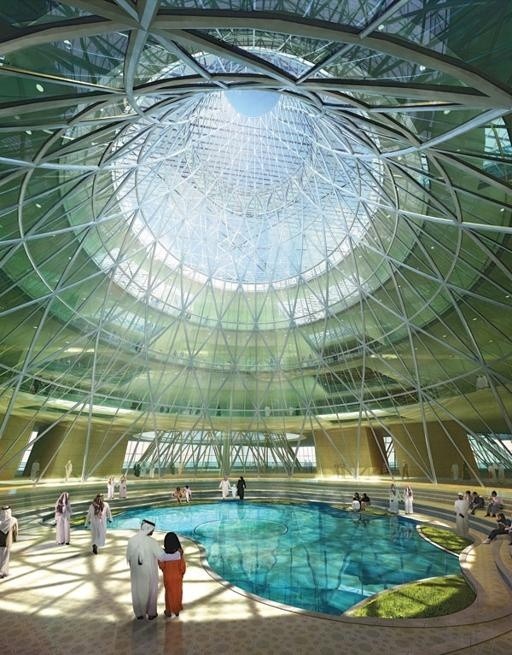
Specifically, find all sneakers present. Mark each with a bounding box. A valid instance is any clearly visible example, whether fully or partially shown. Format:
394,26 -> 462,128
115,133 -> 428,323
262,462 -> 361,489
470,510 -> 497,518
136,612 -> 181,621
482,538 -> 491,544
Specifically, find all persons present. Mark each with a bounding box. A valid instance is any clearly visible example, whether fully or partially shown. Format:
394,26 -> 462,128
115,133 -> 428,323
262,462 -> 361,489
119,475 -> 127,498
131,397 -> 301,416
134,461 -> 247,503
29,459 -> 40,481
84,492 -> 113,554
158,532 -> 186,617
126,516 -> 183,620
347,460 -> 511,543
0,504 -> 19,578
64,459 -> 73,482
106,475 -> 115,500
31,374 -> 54,396
474,373 -> 488,391
55,491 -> 72,544
